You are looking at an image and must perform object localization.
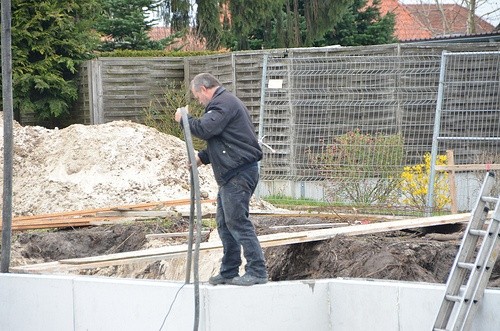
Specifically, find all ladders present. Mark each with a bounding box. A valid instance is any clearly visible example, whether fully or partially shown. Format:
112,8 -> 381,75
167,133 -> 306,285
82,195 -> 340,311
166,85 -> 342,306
431,171 -> 500,330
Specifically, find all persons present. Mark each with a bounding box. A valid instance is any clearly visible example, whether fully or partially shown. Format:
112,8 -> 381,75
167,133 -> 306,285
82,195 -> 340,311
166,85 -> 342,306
174,73 -> 268,286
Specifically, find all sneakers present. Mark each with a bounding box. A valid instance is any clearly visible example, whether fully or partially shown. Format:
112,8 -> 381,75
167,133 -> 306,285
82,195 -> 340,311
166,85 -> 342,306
209,271 -> 240,284
233,271 -> 268,285
